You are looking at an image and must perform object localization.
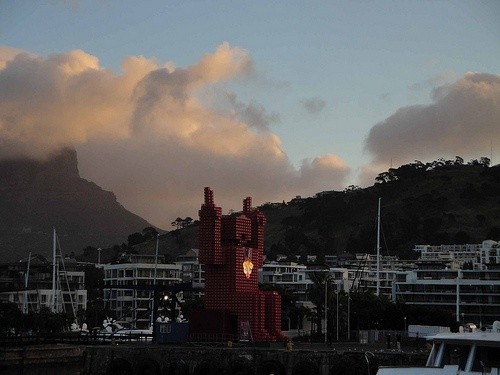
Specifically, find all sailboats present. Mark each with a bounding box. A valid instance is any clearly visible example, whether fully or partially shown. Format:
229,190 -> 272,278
352,195 -> 405,305
5,225 -> 162,343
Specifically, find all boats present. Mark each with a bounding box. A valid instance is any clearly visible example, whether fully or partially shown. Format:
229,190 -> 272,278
375,319 -> 500,375
0,239 -> 499,329
